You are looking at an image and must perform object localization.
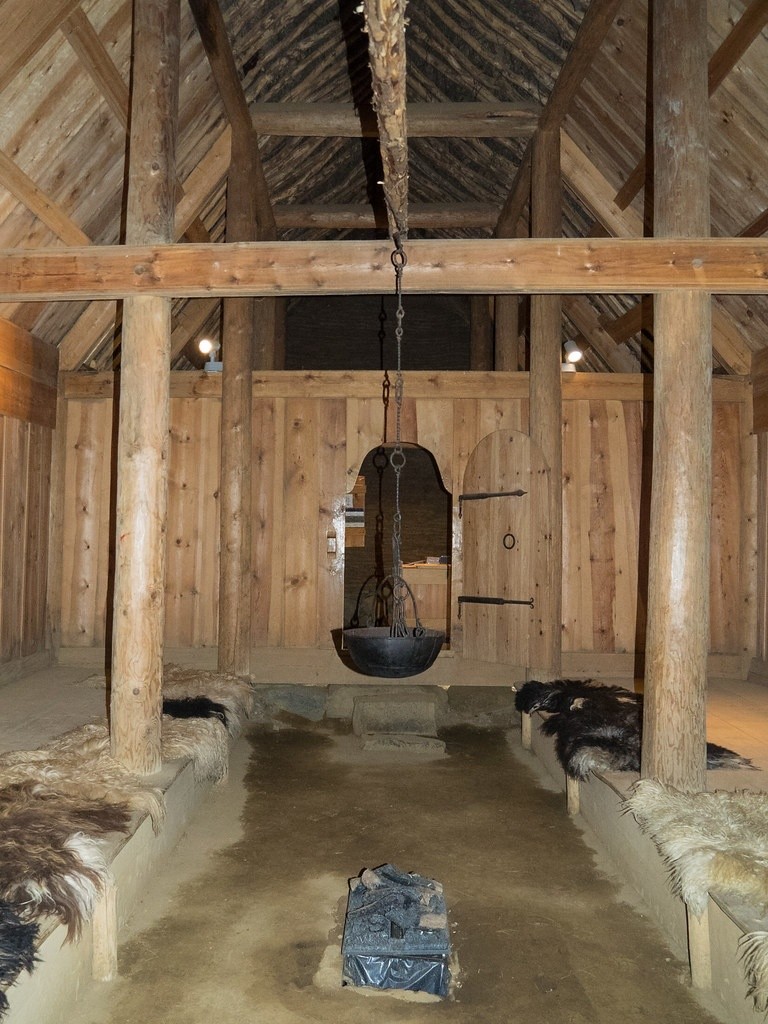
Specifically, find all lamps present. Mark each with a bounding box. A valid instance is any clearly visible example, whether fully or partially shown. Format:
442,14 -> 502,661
562,339 -> 582,373
199,338 -> 224,371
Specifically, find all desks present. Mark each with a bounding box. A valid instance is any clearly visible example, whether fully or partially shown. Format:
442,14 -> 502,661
340,879 -> 452,997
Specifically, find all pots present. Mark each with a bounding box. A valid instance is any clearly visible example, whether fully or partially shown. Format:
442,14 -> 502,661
343,628 -> 445,678
